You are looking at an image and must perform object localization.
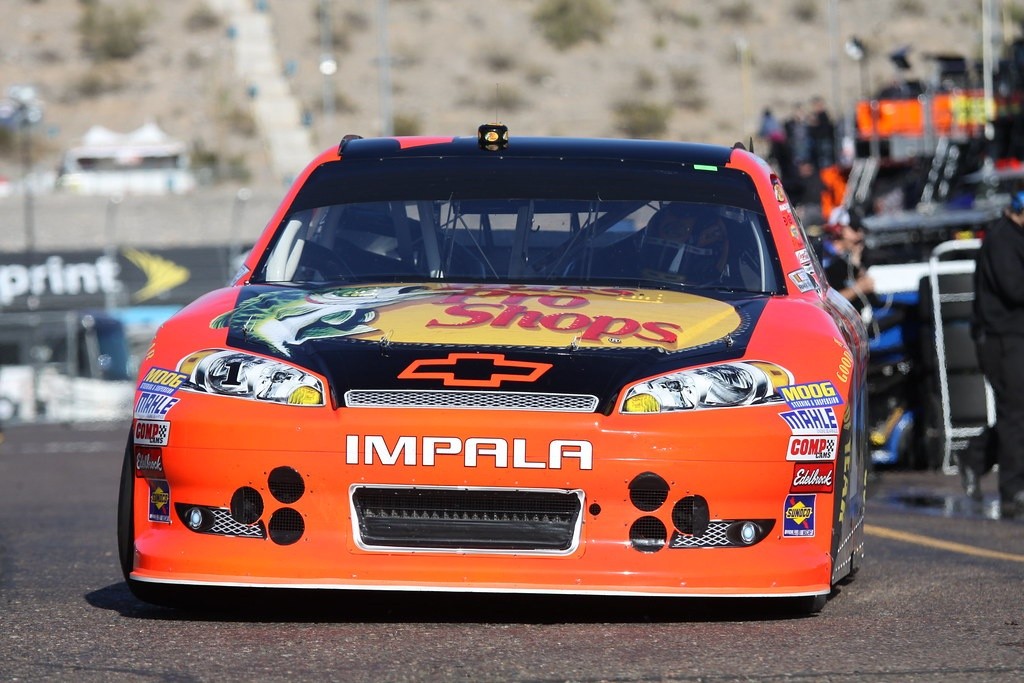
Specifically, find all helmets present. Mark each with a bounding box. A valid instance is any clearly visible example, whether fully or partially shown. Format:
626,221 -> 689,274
639,203 -> 728,286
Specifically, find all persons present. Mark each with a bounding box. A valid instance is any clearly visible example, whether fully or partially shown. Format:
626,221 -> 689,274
759,94 -> 847,224
955,192 -> 1024,523
818,214 -> 882,309
639,203 -> 731,286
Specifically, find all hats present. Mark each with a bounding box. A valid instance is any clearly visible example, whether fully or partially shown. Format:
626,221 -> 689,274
827,204 -> 869,233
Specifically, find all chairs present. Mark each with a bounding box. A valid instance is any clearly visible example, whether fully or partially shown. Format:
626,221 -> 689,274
635,202 -> 734,288
338,211 -> 444,281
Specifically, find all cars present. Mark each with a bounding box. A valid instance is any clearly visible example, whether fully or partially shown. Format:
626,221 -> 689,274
852,80 -> 1024,137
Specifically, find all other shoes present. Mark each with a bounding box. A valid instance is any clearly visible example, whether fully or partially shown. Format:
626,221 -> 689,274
953,449 -> 983,503
998,489 -> 1024,520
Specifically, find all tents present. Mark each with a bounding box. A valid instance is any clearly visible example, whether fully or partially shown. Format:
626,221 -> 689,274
68,122 -> 184,170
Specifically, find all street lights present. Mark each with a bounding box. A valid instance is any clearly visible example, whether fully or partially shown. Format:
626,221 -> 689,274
840,34 -> 872,103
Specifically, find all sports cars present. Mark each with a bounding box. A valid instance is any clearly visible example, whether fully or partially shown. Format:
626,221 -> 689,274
117,133 -> 870,614
